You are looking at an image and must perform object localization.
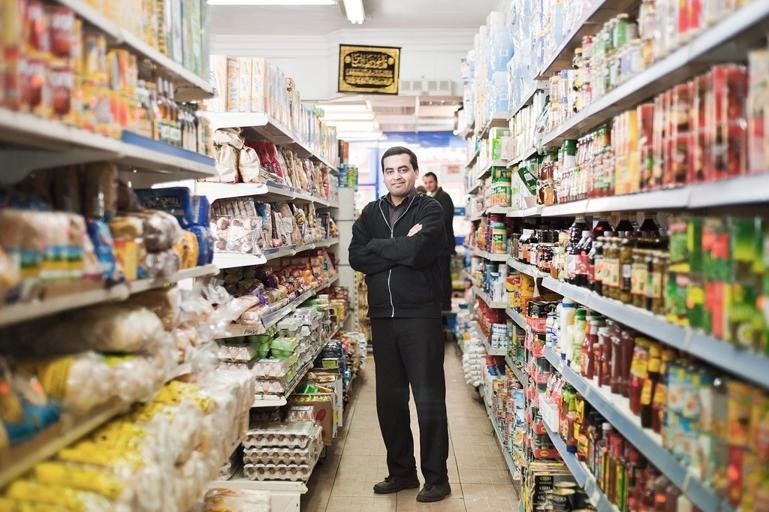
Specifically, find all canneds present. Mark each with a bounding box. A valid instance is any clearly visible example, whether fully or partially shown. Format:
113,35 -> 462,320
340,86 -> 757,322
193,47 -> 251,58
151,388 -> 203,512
532,1 -> 768,134
584,233 -> 670,318
530,62 -> 747,205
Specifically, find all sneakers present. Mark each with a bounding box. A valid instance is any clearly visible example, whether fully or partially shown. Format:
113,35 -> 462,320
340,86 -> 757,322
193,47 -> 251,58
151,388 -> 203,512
372,478 -> 419,494
416,482 -> 451,501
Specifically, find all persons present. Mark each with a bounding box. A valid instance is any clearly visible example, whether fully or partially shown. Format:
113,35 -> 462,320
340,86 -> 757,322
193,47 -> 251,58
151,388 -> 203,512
348,146 -> 451,503
423,172 -> 454,310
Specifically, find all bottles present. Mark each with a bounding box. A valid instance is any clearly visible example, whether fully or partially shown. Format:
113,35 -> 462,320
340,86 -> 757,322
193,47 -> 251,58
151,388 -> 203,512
539,287 -> 769,502
547,366 -> 769,510
745,47 -> 768,181
512,209 -> 658,375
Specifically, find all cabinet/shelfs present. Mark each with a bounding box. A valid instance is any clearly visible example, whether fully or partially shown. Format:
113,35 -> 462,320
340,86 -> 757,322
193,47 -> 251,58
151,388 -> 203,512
448,0 -> 768,511
206,51 -> 368,452
1,0 -> 243,512
